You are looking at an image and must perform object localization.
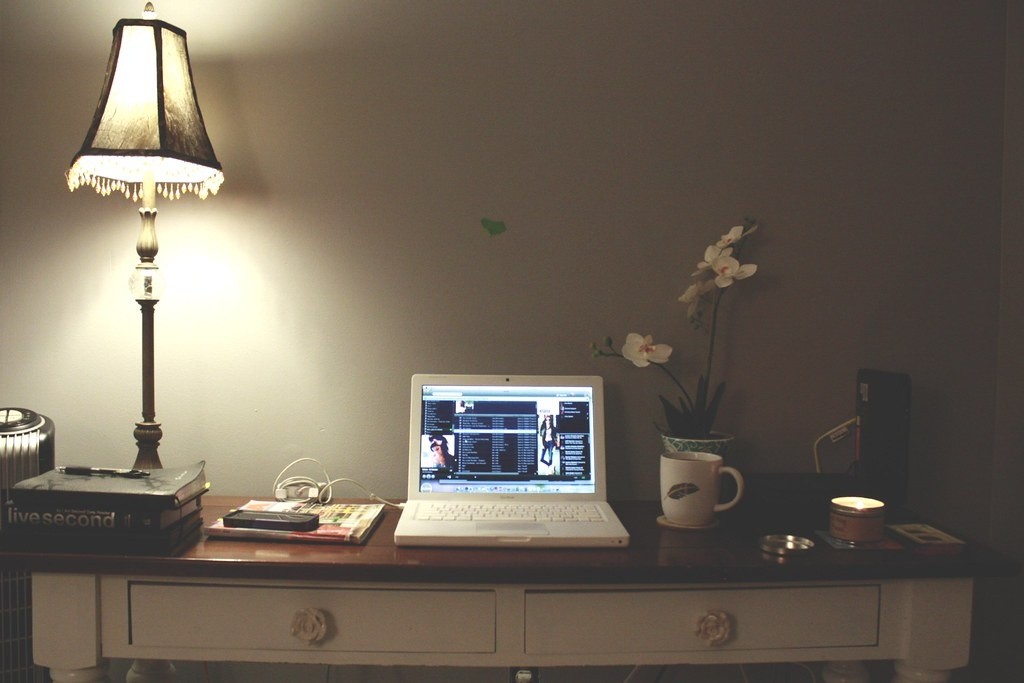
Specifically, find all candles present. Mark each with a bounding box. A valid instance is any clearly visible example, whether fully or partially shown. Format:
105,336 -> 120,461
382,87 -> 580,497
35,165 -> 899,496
829,494 -> 887,545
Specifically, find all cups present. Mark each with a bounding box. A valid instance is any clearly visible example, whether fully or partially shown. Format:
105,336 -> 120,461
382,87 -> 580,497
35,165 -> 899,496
659,451 -> 744,526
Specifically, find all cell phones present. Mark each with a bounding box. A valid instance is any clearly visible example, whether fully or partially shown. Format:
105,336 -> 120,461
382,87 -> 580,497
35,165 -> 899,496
223,509 -> 319,531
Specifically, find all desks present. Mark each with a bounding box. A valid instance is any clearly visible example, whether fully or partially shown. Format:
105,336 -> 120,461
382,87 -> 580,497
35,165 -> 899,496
0,476 -> 1019,683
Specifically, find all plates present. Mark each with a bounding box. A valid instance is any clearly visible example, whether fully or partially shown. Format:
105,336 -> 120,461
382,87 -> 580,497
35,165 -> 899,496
758,534 -> 815,555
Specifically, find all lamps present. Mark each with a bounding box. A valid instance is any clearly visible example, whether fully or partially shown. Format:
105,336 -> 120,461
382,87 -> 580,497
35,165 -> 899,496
65,2 -> 225,469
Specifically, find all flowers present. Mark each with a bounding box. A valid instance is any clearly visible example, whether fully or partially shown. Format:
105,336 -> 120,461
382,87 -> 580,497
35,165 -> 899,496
592,213 -> 759,439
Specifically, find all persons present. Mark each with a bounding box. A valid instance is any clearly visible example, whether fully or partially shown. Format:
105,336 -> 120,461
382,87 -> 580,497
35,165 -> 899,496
540,414 -> 561,476
427,433 -> 456,476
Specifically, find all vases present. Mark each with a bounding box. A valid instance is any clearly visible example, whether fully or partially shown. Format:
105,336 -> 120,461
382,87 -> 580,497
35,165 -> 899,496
661,429 -> 736,467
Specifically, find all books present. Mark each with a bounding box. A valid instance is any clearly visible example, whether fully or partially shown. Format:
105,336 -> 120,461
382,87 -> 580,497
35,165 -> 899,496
204,500 -> 386,544
10,462 -> 209,556
886,522 -> 966,557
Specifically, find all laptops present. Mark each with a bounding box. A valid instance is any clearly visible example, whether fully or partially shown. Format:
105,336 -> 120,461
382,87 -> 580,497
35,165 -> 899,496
394,373 -> 630,547
